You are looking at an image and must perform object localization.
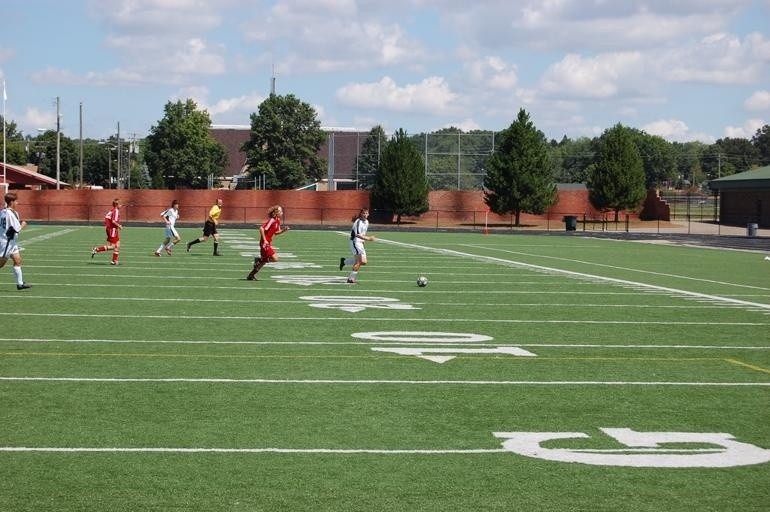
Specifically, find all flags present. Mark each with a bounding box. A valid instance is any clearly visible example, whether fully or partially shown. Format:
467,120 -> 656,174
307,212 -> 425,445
3,81 -> 9,103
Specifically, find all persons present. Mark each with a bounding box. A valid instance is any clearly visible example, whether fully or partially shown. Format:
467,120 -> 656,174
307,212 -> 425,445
0,193 -> 33,290
154,201 -> 182,256
186,198 -> 224,256
339,206 -> 375,282
91,198 -> 125,265
246,206 -> 290,280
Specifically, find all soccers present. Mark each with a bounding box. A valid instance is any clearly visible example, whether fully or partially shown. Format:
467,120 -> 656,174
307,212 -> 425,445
417,277 -> 427,287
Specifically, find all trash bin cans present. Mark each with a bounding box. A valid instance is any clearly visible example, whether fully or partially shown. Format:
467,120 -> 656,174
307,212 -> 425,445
564,216 -> 577,231
747,223 -> 758,236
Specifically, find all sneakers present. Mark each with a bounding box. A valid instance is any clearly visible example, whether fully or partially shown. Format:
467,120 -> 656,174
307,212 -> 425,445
110,261 -> 119,265
254,258 -> 260,272
153,250 -> 161,257
91,246 -> 96,259
164,246 -> 171,255
247,276 -> 259,281
212,251 -> 223,256
347,278 -> 359,284
186,242 -> 190,252
339,257 -> 345,271
17,282 -> 33,290
